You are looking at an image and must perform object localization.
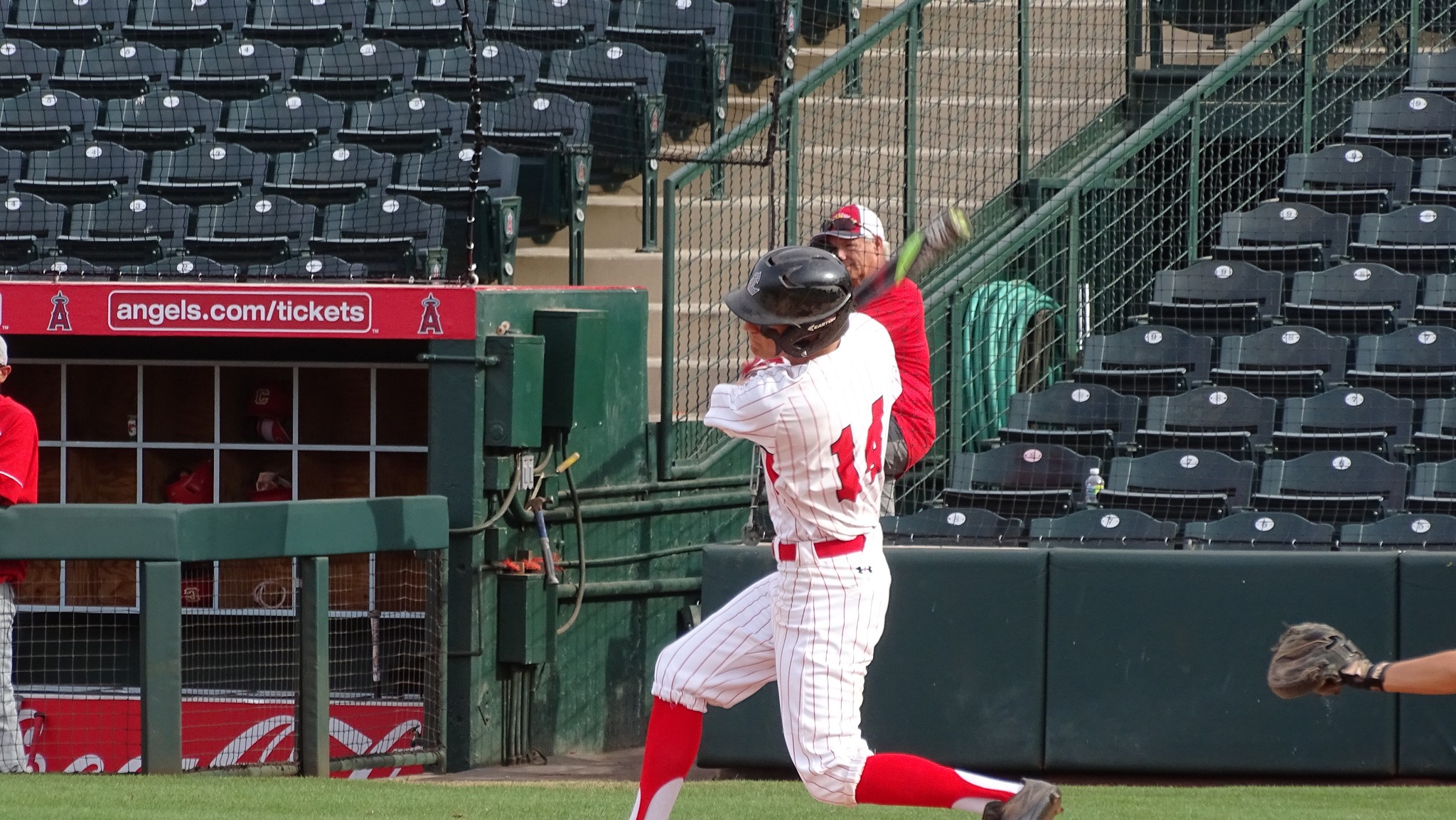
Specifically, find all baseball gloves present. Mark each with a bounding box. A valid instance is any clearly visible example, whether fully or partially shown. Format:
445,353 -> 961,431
1268,622 -> 1367,700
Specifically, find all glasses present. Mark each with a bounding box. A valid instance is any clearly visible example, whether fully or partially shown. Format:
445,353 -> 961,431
822,219 -> 876,238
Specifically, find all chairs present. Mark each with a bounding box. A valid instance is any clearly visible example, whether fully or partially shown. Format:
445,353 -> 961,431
0,0 -> 863,276
880,52 -> 1456,551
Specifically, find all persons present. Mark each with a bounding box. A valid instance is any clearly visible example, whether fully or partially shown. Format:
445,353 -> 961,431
1268,622 -> 1456,699
0,334 -> 38,773
808,205 -> 936,518
628,246 -> 1068,820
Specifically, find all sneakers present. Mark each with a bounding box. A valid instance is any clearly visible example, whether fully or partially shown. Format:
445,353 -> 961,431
977,776 -> 1066,820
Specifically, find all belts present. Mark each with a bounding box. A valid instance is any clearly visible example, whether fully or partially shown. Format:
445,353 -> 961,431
772,534 -> 866,562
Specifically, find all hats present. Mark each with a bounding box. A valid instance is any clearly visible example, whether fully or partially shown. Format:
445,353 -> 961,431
0,336 -> 8,365
809,204 -> 885,249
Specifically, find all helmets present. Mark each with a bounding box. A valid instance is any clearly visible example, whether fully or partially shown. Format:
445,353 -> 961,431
726,246 -> 854,357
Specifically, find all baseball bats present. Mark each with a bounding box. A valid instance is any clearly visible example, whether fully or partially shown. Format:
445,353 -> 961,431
850,207 -> 971,313
